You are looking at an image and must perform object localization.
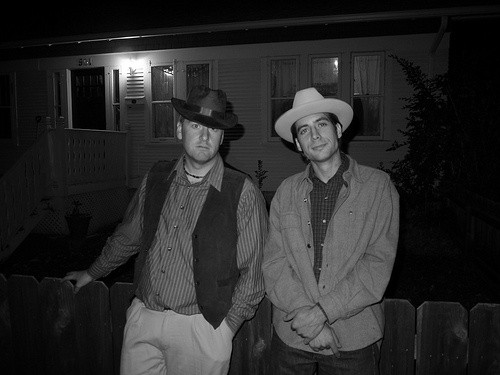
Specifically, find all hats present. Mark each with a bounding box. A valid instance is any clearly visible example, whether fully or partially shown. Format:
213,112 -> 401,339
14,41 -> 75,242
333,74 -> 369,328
172,85 -> 237,129
274,87 -> 354,143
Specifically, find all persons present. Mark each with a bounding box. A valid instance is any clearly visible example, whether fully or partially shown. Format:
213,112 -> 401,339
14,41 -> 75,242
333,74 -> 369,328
261,86 -> 399,375
61,85 -> 268,375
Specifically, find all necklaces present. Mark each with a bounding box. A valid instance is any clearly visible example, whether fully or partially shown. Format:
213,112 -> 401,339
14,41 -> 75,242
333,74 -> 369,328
182,156 -> 205,180
